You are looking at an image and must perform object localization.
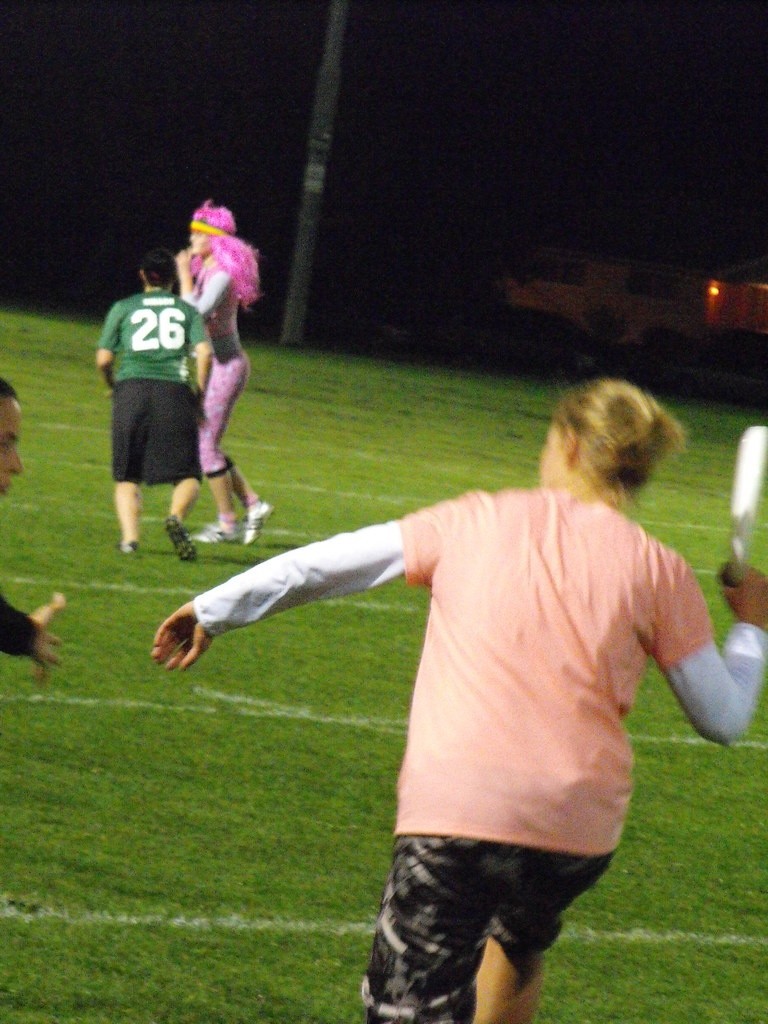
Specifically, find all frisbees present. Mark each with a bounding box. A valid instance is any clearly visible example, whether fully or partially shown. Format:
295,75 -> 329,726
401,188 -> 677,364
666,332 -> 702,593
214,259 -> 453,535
720,423 -> 768,588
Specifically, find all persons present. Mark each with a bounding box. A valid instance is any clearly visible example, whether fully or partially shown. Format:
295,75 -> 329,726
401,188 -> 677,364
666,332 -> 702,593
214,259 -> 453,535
0,377 -> 67,685
173,199 -> 274,546
149,377 -> 767,1024
97,246 -> 214,561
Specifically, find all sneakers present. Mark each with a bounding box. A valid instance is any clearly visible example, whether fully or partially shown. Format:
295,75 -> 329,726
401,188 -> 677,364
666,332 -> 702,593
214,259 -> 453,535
189,525 -> 242,543
243,501 -> 274,545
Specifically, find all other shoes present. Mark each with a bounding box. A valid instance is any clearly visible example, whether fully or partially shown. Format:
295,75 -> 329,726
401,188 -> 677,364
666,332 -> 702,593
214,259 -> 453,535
113,541 -> 136,556
163,518 -> 196,561
